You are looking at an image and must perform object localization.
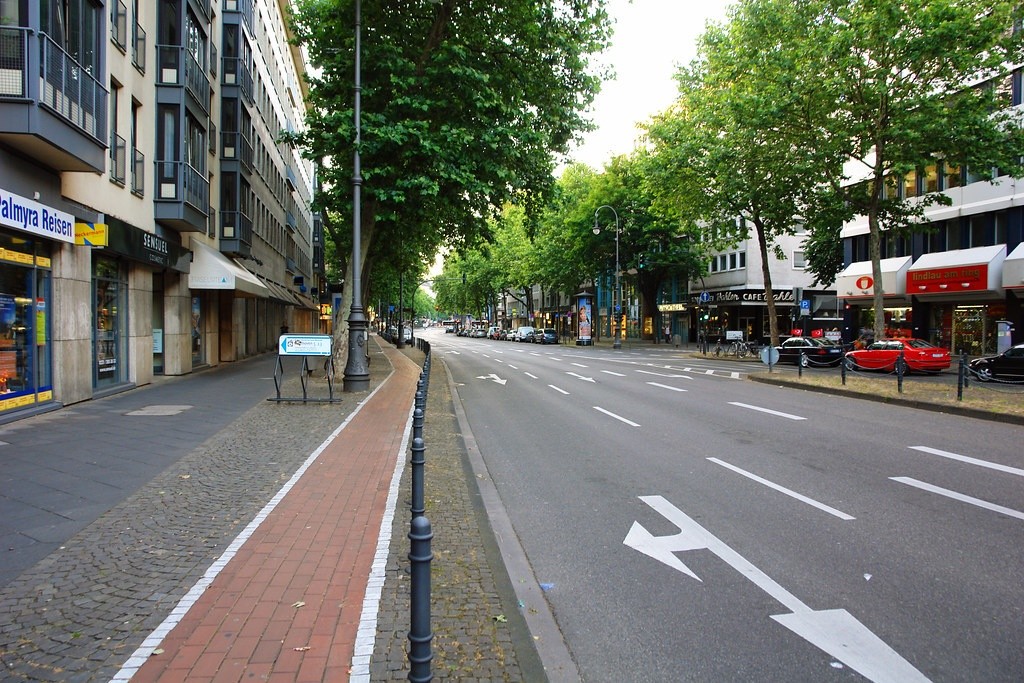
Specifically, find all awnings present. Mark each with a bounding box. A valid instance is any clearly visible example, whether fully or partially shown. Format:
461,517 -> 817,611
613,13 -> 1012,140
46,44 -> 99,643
837,256 -> 912,299
189,236 -> 270,299
258,277 -> 320,312
905,244 -> 1005,300
1002,243 -> 1024,290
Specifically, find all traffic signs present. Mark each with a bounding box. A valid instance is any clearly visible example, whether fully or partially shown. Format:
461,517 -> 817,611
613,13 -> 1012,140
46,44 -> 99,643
278,333 -> 332,355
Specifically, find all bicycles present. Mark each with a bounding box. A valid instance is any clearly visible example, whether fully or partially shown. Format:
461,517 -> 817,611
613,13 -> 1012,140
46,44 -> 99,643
848,338 -> 870,350
711,336 -> 753,359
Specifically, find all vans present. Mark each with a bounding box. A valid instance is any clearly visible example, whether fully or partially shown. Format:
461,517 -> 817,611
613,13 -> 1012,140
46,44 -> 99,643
515,326 -> 536,342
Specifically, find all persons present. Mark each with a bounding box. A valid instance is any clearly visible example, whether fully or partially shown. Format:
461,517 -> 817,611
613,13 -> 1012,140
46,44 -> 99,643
579,307 -> 590,327
665,325 -> 671,343
280,322 -> 289,334
864,326 -> 874,344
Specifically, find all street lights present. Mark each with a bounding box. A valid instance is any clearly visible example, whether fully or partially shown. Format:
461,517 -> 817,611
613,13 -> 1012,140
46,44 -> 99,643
592,205 -> 622,349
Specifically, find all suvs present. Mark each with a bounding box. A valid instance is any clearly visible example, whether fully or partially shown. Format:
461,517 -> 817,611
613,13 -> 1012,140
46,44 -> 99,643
533,327 -> 559,345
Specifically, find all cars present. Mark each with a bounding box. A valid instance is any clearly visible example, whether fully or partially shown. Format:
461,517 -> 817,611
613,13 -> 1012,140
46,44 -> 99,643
388,317 -> 441,343
525,330 -> 536,343
969,341 -> 1024,383
843,336 -> 952,377
773,334 -> 846,368
443,316 -> 517,342
